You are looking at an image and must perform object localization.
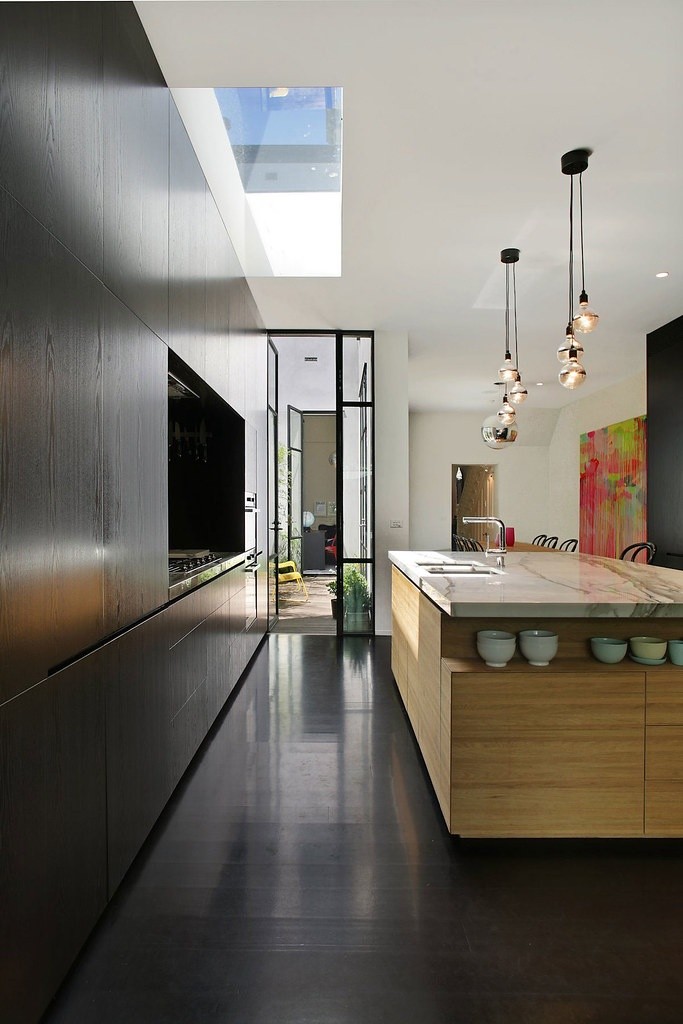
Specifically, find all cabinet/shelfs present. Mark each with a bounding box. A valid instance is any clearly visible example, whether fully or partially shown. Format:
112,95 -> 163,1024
391,564 -> 683,838
0,1 -> 268,1024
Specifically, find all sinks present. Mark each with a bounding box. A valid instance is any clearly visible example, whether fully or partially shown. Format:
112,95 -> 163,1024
415,562 -> 478,566
427,570 -> 497,575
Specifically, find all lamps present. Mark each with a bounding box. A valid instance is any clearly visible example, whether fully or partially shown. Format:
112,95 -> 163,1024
328,450 -> 336,466
481,247 -> 527,449
556,150 -> 599,389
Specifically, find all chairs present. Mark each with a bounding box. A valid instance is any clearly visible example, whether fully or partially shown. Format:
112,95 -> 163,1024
452,534 -> 657,564
277,560 -> 309,604
325,534 -> 337,562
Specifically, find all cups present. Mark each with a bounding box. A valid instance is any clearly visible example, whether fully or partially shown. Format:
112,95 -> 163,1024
506,527 -> 515,547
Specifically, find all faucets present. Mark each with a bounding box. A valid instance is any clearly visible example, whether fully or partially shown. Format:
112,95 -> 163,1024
463,516 -> 507,554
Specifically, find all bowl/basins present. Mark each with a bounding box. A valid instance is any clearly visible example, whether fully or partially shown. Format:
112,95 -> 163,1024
520,630 -> 559,643
478,631 -> 516,645
478,645 -> 516,667
668,640 -> 683,665
591,638 -> 627,663
520,643 -> 558,666
630,637 -> 667,659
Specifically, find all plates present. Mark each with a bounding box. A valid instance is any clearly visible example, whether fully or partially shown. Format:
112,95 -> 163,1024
629,654 -> 666,665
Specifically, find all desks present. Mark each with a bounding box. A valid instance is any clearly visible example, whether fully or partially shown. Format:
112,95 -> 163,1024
458,540 -> 565,552
303,530 -> 326,570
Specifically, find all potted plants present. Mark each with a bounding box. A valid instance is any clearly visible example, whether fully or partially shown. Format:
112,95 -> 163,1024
327,580 -> 346,619
343,567 -> 372,632
